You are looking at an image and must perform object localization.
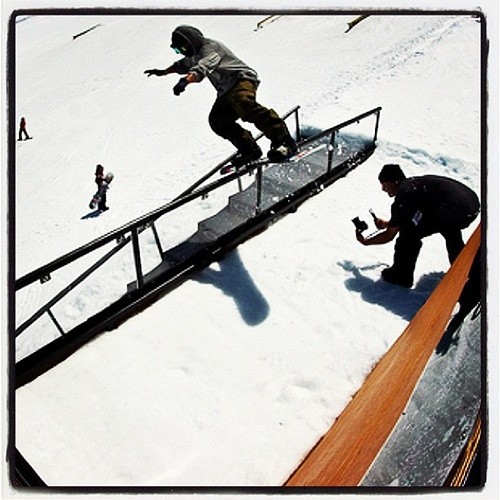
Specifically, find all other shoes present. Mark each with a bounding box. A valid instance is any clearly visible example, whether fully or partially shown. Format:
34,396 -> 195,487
99,206 -> 109,211
382,268 -> 414,288
232,146 -> 262,165
266,139 -> 297,161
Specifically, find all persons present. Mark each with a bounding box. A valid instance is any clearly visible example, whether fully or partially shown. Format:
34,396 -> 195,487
144,25 -> 298,167
89,165 -> 112,212
17,117 -> 32,140
356,164 -> 480,288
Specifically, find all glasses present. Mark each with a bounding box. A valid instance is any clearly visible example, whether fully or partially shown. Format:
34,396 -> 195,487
174,46 -> 186,54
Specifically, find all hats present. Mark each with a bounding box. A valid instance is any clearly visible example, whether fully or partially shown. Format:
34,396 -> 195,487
379,163 -> 402,181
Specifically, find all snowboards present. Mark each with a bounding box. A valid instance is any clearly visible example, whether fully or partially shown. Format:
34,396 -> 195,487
220,150 -> 308,175
89,173 -> 114,209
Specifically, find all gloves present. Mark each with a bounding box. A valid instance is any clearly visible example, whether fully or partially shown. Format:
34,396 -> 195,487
173,78 -> 187,96
145,69 -> 165,77
